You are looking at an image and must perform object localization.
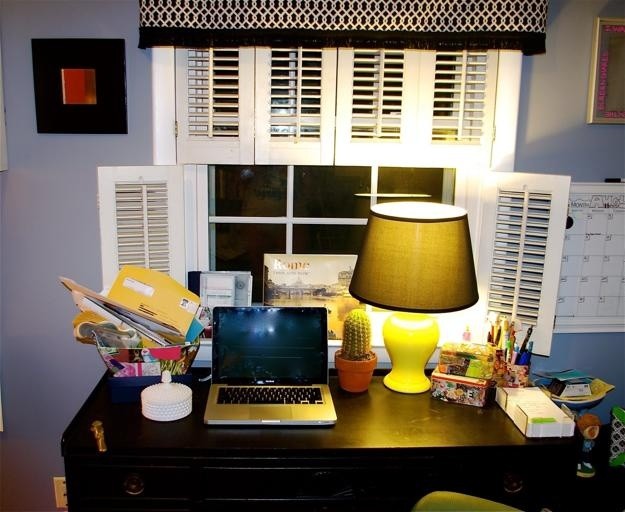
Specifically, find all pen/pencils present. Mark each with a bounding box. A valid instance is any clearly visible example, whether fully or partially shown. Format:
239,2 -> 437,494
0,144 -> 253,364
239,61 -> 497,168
487,312 -> 534,365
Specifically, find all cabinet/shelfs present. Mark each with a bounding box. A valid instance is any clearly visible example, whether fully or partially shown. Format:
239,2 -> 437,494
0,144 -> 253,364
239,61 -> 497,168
60,363 -> 585,511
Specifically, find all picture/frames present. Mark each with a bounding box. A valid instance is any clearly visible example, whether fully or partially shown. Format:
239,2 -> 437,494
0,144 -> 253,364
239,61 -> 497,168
31,37 -> 127,134
586,17 -> 625,125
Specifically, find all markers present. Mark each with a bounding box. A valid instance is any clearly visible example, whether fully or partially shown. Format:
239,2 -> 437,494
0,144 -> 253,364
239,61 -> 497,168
604,177 -> 625,183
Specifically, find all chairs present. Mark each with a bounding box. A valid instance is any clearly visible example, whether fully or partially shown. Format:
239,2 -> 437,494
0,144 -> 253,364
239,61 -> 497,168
413,490 -> 523,512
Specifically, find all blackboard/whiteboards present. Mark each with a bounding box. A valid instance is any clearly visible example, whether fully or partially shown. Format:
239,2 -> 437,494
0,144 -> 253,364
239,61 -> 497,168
552,182 -> 625,334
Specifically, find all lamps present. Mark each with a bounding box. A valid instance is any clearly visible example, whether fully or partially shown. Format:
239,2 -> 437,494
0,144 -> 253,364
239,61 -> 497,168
346,201 -> 480,393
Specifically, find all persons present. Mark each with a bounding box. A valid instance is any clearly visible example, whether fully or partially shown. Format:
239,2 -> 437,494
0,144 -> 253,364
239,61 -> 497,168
576,413 -> 600,478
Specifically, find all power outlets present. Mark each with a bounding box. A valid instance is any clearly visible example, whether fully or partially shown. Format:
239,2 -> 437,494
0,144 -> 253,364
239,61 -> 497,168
54,477 -> 68,508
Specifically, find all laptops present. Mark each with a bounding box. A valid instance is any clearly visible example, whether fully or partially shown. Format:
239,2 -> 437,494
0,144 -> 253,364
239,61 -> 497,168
204,306 -> 337,426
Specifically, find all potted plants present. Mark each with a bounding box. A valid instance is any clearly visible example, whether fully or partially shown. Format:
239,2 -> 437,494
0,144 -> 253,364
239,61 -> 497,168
334,308 -> 378,392
